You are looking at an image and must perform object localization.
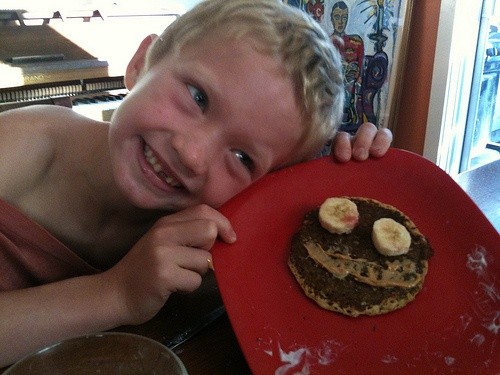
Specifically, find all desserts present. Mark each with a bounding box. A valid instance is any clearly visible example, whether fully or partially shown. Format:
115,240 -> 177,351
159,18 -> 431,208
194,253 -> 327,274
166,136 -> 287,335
287,195 -> 429,317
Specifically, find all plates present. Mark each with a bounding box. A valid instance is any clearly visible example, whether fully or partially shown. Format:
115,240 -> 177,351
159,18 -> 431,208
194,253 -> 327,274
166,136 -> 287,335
207,148 -> 500,375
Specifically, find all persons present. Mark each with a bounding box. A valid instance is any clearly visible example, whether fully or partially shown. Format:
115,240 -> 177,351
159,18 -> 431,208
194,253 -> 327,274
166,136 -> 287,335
0,1 -> 395,375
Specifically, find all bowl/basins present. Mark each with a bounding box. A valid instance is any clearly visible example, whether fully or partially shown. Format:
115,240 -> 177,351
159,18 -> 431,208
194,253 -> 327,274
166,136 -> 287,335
2,332 -> 191,375
